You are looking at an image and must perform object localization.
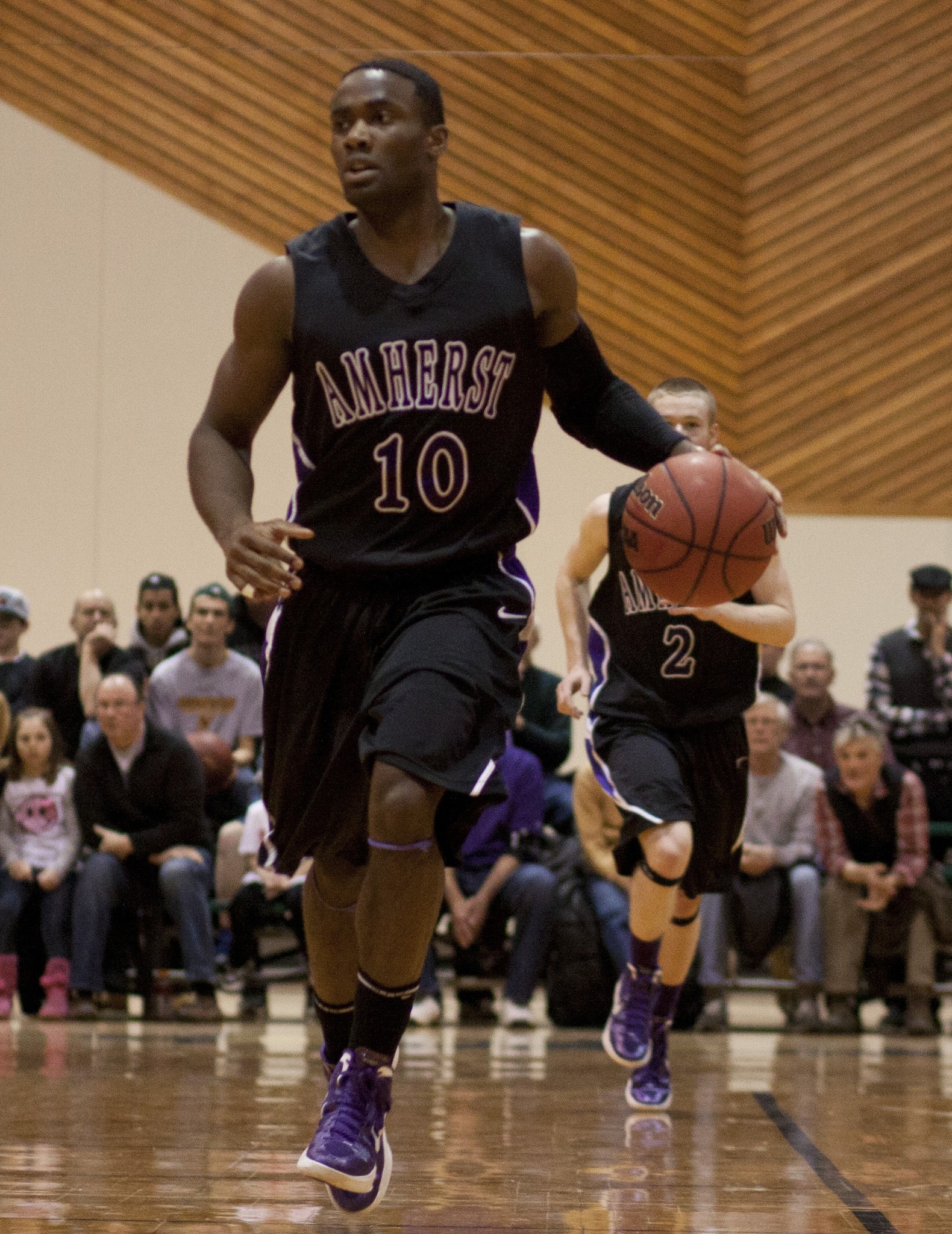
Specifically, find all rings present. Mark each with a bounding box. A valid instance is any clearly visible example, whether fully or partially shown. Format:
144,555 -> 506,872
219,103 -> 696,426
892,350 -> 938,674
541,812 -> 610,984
242,584 -> 254,598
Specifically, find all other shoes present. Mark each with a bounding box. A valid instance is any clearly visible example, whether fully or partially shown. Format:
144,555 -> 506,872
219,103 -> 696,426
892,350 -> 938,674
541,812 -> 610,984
695,983 -> 939,1038
0,954 -> 271,1023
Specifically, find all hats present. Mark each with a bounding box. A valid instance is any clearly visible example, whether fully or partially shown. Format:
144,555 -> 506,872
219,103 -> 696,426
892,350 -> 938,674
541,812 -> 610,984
195,582 -> 229,600
139,573 -> 174,591
909,565 -> 951,594
0,587 -> 29,620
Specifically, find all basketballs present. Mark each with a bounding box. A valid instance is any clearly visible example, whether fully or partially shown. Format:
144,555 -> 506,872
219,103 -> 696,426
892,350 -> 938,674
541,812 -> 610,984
185,729 -> 236,791
619,449 -> 780,609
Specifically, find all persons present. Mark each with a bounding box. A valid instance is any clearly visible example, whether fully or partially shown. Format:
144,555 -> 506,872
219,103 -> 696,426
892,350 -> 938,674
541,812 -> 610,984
556,377 -> 797,1112
187,58 -> 787,1215
0,565 -> 952,1031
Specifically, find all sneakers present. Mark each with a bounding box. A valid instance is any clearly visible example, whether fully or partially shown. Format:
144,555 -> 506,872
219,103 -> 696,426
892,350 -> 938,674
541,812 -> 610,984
295,1047 -> 393,1189
621,1013 -> 674,1111
320,1045 -> 400,1215
602,962 -> 661,1066
